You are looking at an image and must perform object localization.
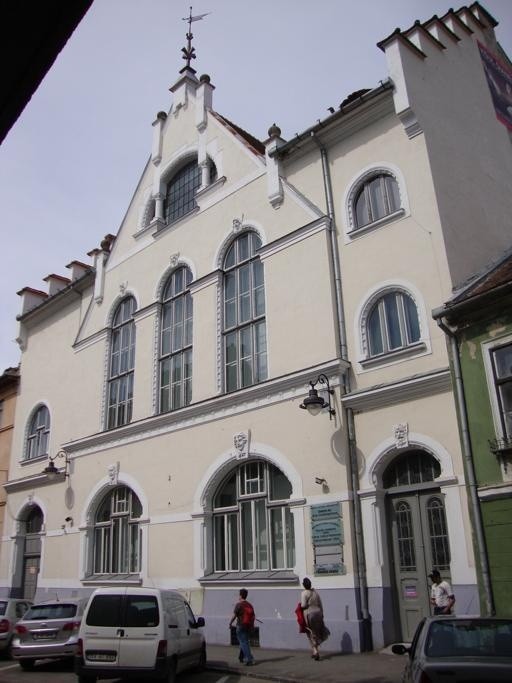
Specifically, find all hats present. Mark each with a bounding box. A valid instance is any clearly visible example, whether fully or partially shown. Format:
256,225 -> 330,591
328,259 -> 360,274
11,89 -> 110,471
428,571 -> 440,577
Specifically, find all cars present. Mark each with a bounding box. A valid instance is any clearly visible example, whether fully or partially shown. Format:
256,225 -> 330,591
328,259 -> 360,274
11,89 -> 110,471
0,586 -> 206,683
391,615 -> 512,683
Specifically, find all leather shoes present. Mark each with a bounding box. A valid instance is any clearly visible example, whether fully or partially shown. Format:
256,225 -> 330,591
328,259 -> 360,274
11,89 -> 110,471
312,653 -> 320,661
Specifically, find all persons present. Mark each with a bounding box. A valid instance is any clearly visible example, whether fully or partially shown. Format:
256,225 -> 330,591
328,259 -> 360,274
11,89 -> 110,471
297,577 -> 330,660
229,589 -> 257,666
427,570 -> 454,615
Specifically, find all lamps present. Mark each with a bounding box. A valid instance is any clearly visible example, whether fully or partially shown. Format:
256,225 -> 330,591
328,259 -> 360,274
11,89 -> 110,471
42,451 -> 68,481
299,373 -> 332,420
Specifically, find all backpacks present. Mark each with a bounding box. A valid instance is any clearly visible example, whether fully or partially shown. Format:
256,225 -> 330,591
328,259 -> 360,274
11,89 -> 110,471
242,606 -> 255,625
